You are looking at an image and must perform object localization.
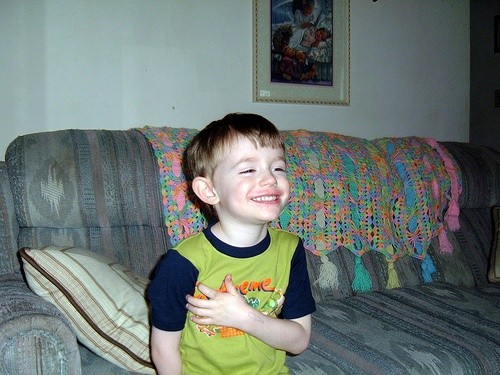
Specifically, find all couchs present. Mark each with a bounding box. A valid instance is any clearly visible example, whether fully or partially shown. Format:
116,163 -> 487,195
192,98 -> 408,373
0,125 -> 500,375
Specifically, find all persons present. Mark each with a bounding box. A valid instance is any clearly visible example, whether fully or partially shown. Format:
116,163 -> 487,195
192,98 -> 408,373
148,112 -> 316,375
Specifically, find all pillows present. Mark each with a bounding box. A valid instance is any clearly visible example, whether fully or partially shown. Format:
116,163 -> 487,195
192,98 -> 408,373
487,205 -> 500,283
19,244 -> 158,375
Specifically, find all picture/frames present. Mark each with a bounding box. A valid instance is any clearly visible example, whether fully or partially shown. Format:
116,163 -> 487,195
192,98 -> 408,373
252,0 -> 350,107
